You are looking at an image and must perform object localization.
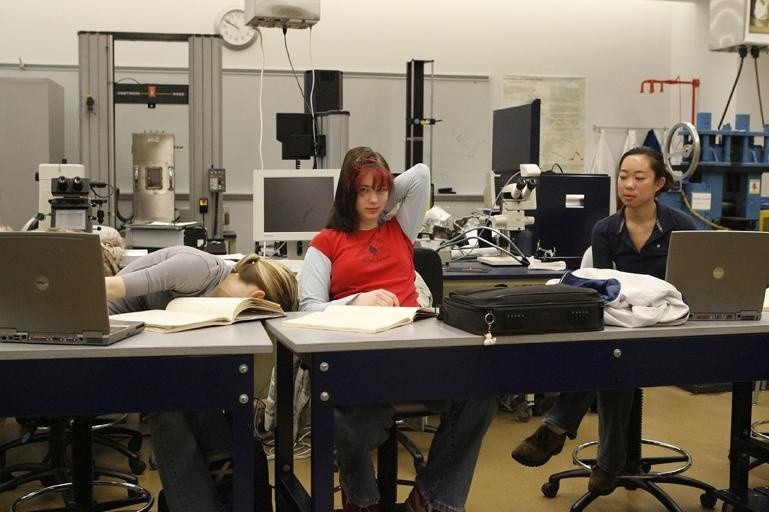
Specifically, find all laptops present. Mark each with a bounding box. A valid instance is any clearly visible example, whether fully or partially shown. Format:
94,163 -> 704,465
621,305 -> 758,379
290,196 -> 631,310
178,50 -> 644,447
0,232 -> 146,347
663,229 -> 769,321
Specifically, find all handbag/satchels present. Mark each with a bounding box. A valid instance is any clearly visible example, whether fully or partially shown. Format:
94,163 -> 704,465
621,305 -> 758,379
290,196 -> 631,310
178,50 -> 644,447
436,284 -> 606,337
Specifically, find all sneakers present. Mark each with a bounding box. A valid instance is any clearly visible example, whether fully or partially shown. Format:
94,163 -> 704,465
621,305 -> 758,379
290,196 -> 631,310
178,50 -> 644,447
587,463 -> 619,497
404,483 -> 438,512
510,424 -> 567,468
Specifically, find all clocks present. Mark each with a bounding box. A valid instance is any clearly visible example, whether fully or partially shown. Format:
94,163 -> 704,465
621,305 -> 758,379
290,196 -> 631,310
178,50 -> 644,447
219,10 -> 260,50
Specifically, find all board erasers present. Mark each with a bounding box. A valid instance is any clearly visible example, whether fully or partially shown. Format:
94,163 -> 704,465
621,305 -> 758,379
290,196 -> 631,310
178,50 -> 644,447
438,188 -> 457,194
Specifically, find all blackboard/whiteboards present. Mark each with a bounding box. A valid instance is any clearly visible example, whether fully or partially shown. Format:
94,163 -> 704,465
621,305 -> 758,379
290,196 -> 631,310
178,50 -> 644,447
0,64 -> 493,201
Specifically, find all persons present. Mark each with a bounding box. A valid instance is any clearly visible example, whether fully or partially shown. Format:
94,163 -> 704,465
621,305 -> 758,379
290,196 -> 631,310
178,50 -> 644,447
298,147 -> 499,512
105,246 -> 297,512
511,146 -> 697,495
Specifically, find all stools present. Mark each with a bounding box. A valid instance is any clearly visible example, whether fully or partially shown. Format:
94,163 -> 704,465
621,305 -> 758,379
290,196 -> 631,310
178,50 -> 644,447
2,399 -> 154,512
542,381 -> 720,511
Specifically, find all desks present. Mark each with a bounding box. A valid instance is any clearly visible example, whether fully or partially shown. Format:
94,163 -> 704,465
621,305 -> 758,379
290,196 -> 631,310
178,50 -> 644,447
1,308 -> 274,511
263,304 -> 769,512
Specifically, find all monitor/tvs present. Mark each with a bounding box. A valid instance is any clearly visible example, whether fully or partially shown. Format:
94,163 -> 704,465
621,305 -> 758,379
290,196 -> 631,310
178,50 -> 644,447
252,168 -> 342,241
51,204 -> 92,233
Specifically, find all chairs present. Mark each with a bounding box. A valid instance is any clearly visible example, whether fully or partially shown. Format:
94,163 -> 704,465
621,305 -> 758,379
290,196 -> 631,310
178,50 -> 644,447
325,247 -> 455,506
